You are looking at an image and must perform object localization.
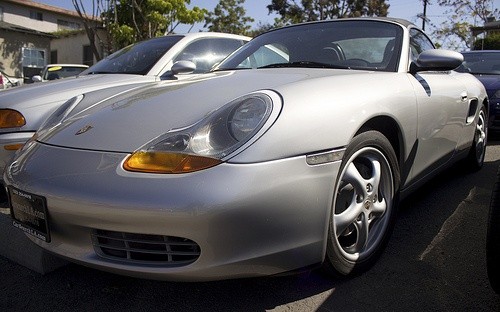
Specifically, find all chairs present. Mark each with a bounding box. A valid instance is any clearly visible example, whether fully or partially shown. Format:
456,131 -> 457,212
315,43 -> 345,62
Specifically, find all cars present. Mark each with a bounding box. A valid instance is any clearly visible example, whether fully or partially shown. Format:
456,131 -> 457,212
32,63 -> 94,85
0,32 -> 290,201
457,49 -> 500,134
9,17 -> 491,282
